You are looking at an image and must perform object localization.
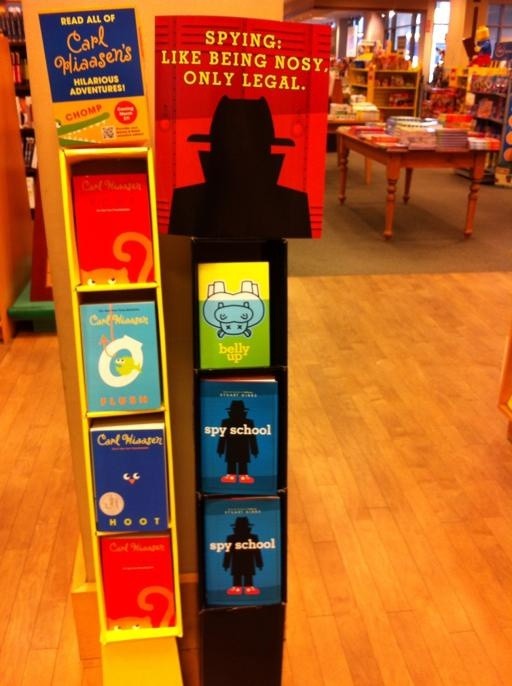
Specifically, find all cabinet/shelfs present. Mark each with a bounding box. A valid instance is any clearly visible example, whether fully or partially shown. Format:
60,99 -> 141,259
2,2 -> 339,685
283,3 -> 511,260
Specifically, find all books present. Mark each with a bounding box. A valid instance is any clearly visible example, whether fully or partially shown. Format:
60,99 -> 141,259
1,14 -> 36,208
200,378 -> 279,496
203,498 -> 280,605
329,77 -> 505,169
80,301 -> 161,412
197,261 -> 270,370
73,174 -> 154,285
90,419 -> 168,532
100,534 -> 177,629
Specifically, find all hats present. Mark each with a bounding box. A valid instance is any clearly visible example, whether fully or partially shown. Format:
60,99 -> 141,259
189,95 -> 297,146
